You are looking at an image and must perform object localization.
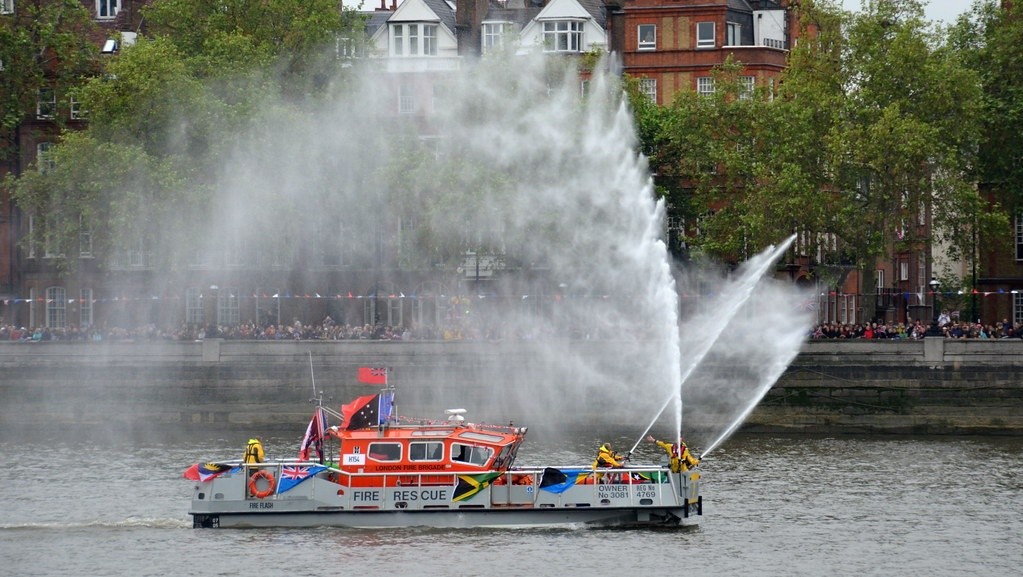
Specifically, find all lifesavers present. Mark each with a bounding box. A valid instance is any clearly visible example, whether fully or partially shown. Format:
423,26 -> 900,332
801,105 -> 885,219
248,469 -> 276,499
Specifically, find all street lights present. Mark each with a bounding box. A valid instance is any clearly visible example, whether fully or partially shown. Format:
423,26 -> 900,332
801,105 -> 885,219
926,276 -> 943,337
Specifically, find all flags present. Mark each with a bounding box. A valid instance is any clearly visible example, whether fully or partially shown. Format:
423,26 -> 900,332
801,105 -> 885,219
539,467 -> 591,494
452,471 -> 505,504
182,462 -> 232,482
301,368 -> 394,461
277,464 -> 329,495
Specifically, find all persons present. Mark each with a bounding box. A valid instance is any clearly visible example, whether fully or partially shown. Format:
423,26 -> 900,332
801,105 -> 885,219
432,443 -> 481,463
0,311 -> 555,342
591,443 -> 627,483
647,435 -> 700,473
804,316 -> 1023,339
242,436 -> 264,477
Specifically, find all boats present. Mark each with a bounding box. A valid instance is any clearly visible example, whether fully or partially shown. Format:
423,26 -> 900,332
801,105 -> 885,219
182,350 -> 707,531
